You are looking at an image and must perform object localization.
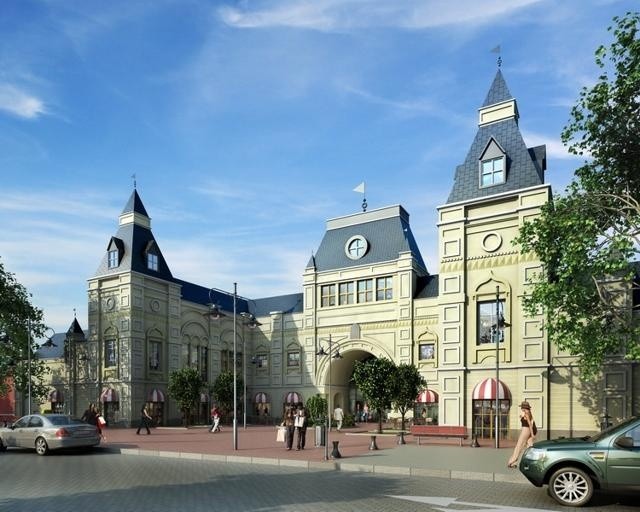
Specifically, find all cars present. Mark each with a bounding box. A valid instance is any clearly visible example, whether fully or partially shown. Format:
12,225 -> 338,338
519,413 -> 640,507
0,413 -> 103,456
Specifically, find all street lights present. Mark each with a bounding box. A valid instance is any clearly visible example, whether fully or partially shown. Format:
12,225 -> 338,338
314,330 -> 346,434
202,281 -> 263,452
486,283 -> 515,450
65,336 -> 91,415
0,316 -> 59,413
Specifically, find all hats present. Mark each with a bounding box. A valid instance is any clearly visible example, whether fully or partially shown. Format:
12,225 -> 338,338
518,402 -> 531,409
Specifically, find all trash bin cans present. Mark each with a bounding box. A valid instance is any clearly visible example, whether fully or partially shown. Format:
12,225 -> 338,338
314,418 -> 326,447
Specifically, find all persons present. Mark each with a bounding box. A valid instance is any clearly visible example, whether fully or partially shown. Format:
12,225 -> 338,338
333,403 -> 345,431
280,404 -> 295,451
135,403 -> 153,435
507,401 -> 537,469
90,407 -> 106,441
80,402 -> 95,423
293,401 -> 310,451
208,405 -> 222,433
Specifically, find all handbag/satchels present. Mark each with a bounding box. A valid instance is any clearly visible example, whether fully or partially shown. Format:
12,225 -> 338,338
277,430 -> 286,442
294,417 -> 304,428
97,416 -> 107,428
